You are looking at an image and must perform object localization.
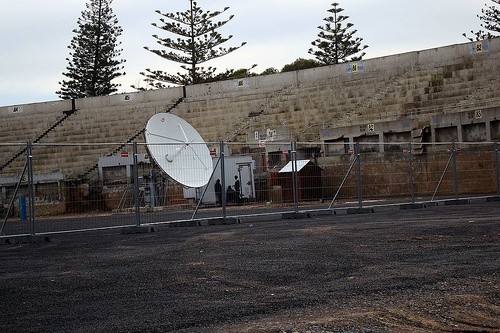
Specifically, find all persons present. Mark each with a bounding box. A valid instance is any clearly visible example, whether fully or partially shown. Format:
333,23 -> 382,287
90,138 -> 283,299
227,175 -> 242,204
215,179 -> 222,207
82,180 -> 91,208
